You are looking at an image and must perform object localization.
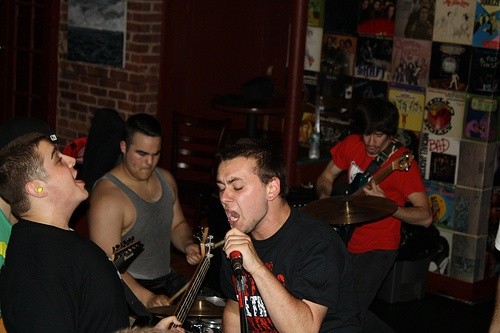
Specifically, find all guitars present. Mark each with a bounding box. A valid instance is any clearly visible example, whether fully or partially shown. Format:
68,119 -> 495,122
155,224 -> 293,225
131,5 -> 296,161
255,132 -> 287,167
337,152 -> 416,198
131,226 -> 216,333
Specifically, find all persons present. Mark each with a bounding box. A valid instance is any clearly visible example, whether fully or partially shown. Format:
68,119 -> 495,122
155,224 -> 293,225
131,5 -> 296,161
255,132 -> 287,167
393,61 -> 419,87
89,113 -> 202,309
0,132 -> 185,333
214,138 -> 363,333
317,97 -> 432,333
321,36 -> 354,73
361,0 -> 395,37
403,0 -> 434,41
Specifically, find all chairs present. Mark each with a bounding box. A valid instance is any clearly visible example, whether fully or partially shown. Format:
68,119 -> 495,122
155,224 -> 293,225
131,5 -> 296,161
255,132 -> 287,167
171,113 -> 237,235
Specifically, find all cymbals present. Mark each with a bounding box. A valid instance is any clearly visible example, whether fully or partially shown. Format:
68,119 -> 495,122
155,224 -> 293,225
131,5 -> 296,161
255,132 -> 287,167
144,298 -> 226,319
301,194 -> 398,225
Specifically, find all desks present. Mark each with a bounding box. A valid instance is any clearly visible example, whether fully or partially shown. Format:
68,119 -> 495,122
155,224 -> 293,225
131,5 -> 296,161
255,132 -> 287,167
212,97 -> 285,148
